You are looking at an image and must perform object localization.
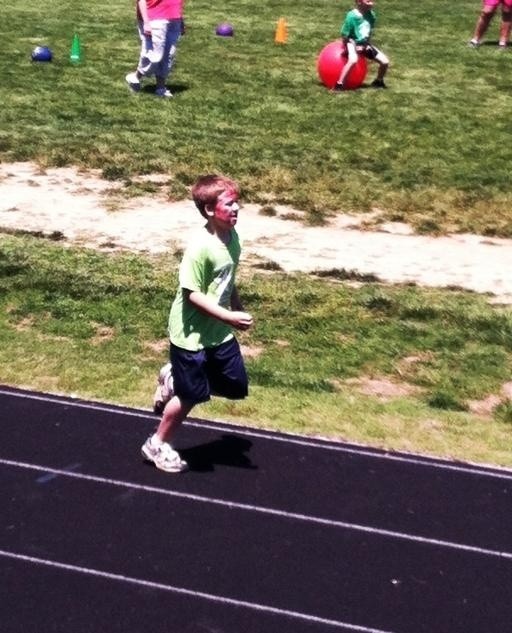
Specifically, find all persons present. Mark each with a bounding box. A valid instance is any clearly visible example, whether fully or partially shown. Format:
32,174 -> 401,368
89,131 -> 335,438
469,0 -> 512,48
126,0 -> 184,99
334,0 -> 390,94
141,175 -> 254,473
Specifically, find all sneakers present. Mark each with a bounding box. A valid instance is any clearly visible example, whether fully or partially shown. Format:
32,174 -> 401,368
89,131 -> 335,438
156,88 -> 173,97
152,362 -> 176,416
334,81 -> 342,91
141,435 -> 189,474
371,79 -> 386,88
467,41 -> 481,49
498,40 -> 507,48
126,73 -> 143,94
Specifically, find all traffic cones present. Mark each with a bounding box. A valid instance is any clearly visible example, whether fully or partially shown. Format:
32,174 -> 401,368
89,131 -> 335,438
275,17 -> 289,43
70,31 -> 82,61
